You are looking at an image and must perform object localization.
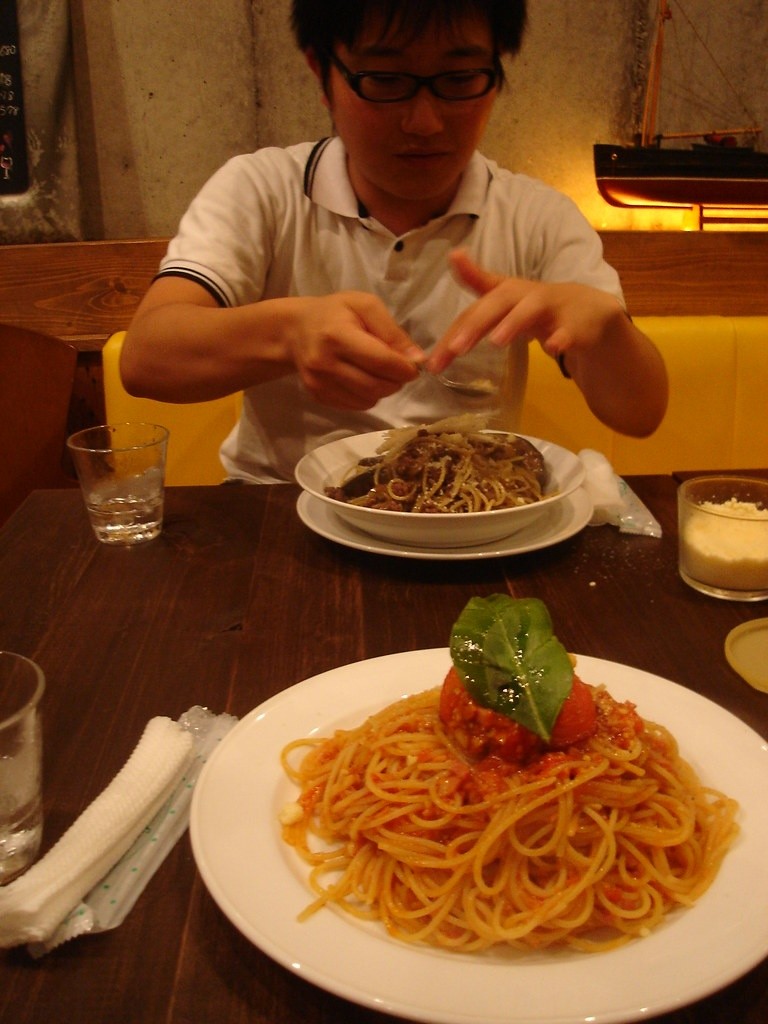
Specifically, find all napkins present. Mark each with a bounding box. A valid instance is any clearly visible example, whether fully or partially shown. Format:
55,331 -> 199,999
0,715 -> 197,948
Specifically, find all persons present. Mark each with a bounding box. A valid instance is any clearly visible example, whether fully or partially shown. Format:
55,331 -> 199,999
118,0 -> 668,486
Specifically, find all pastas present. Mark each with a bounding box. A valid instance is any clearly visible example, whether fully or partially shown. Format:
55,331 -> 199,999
340,427 -> 559,513
281,675 -> 740,954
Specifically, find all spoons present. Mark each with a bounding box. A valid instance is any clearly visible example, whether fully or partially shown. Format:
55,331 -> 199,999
420,367 -> 491,396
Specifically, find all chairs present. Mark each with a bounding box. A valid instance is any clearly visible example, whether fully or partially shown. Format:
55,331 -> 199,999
0,322 -> 78,529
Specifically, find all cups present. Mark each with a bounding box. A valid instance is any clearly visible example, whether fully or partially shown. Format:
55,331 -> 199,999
0,651 -> 44,885
677,476 -> 768,601
67,422 -> 170,545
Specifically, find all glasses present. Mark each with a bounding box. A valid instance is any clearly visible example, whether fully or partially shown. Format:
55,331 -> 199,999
321,42 -> 504,104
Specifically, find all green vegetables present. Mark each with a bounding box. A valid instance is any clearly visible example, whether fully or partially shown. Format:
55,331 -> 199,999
448,593 -> 572,741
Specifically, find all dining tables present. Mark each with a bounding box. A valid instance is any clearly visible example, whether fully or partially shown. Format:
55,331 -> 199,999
0,469 -> 767,1023
674,469 -> 767,621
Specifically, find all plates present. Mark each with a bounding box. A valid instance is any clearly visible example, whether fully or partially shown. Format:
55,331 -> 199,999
191,646 -> 768,1024
297,489 -> 593,560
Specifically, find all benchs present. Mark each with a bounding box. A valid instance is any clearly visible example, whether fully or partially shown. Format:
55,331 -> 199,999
103,315 -> 768,487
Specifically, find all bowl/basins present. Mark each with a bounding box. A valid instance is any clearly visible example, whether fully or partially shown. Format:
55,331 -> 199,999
294,429 -> 586,548
